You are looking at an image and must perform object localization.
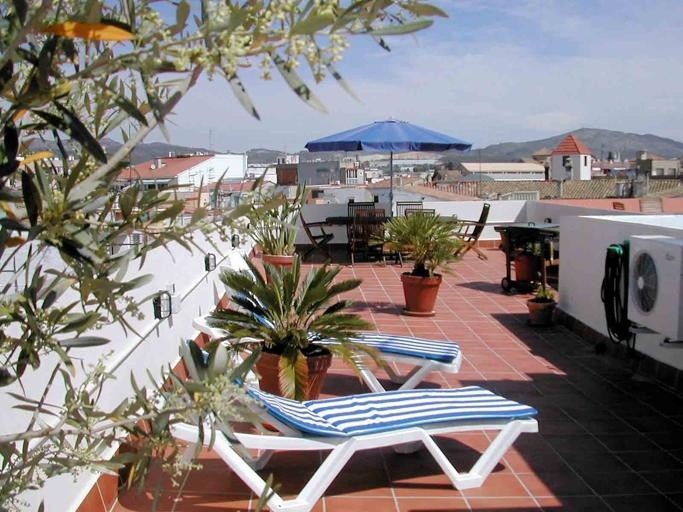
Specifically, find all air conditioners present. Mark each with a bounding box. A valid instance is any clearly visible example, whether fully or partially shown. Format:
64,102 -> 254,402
627,236 -> 683,348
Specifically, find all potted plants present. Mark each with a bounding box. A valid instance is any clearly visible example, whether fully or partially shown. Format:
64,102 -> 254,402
367,213 -> 471,317
204,253 -> 391,400
238,179 -> 311,285
526,285 -> 557,327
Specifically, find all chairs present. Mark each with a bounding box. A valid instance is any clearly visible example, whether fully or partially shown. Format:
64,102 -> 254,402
153,341 -> 539,512
192,291 -> 462,392
296,202 -> 492,267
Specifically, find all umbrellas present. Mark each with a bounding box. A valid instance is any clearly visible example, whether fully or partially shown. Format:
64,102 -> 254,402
303,116 -> 473,260
